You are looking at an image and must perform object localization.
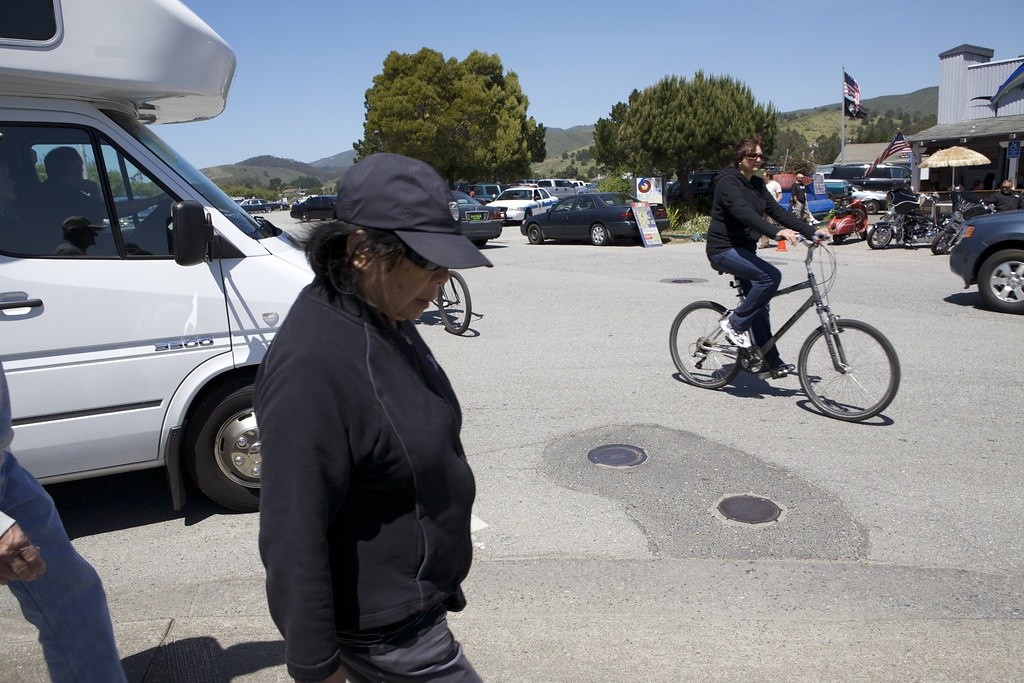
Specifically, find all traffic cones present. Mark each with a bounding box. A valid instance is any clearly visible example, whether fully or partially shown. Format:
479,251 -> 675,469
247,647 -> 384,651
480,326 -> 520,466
775,240 -> 788,252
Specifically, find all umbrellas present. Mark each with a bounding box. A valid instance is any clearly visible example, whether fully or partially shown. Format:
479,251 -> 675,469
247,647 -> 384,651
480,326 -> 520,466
917,146 -> 994,169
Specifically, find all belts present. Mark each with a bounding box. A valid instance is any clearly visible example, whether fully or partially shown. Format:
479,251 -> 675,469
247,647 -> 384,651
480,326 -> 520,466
334,600 -> 448,656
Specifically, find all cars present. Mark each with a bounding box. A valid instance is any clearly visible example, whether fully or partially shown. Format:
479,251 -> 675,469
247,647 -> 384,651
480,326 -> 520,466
668,173 -> 718,212
512,179 -> 539,195
290,195 -> 338,223
520,192 -> 670,245
463,185 -> 513,202
233,199 -> 244,205
564,181 -> 585,189
238,199 -> 281,213
114,196 -> 153,223
485,187 -> 558,223
949,210 -> 1024,316
452,191 -> 503,245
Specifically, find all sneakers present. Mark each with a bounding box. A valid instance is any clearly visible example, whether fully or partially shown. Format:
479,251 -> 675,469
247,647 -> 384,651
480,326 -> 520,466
721,319 -> 752,348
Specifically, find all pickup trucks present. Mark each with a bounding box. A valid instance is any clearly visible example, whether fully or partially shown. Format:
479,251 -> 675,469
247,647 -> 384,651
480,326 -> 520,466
823,180 -> 851,199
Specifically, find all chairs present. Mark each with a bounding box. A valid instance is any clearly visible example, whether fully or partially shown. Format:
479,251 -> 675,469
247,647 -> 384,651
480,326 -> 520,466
586,202 -> 595,209
606,201 -> 614,206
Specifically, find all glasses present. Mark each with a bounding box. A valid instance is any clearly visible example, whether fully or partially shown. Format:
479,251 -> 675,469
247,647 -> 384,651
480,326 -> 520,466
402,247 -> 441,271
745,152 -> 764,160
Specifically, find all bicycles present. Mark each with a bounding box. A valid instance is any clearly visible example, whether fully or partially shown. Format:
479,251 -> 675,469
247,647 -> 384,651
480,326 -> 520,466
669,233 -> 901,422
431,271 -> 472,334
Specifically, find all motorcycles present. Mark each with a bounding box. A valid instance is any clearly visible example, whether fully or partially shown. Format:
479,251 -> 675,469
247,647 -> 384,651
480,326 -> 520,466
828,185 -> 996,255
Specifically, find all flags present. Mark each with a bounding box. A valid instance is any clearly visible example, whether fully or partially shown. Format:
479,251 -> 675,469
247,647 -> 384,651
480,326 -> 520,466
862,129 -> 912,179
844,71 -> 869,118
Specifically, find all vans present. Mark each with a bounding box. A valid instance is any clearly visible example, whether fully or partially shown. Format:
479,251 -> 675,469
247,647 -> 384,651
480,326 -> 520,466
537,179 -> 578,199
829,164 -> 913,215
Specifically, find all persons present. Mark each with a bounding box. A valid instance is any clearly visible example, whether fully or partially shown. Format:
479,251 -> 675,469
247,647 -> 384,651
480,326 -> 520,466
791,173 -> 808,240
0,355 -> 131,683
252,151 -> 497,683
33,146 -> 173,257
705,137 -> 830,380
988,179 -> 1024,215
757,170 -> 783,250
53,216 -> 106,257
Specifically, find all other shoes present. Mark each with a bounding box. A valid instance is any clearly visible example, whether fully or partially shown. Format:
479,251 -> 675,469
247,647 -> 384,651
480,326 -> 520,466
813,220 -> 821,224
809,222 -> 812,226
758,244 -> 769,249
771,364 -> 796,379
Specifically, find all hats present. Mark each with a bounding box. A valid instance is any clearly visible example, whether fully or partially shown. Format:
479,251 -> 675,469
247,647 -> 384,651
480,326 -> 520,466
334,152 -> 494,270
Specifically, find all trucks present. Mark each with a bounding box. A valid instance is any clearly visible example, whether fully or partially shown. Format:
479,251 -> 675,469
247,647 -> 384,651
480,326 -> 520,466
0,0 -> 316,514
